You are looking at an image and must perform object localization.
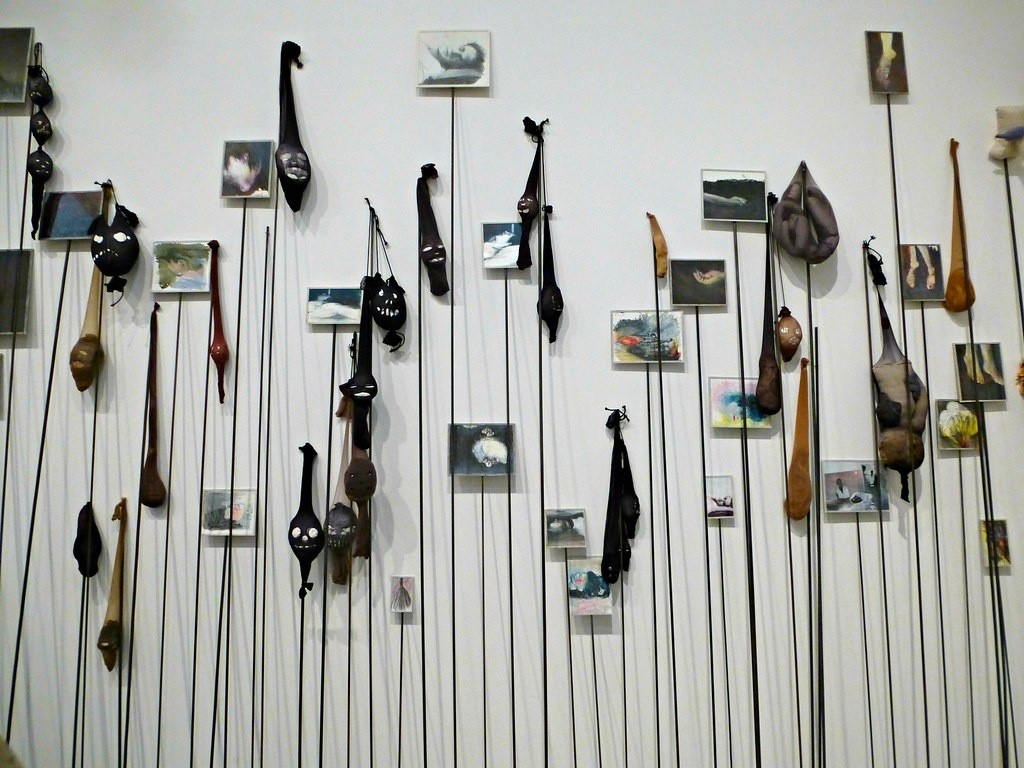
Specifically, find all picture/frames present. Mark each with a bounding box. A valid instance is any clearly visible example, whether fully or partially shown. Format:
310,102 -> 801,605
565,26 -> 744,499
702,474 -> 736,520
864,30 -> 910,95
218,139 -> 275,199
707,375 -> 773,430
201,487 -> 258,537
900,243 -> 946,302
415,29 -> 492,89
447,422 -> 517,477
544,508 -> 589,549
669,257 -> 728,307
700,168 -> 769,223
934,398 -> 980,452
480,221 -> 522,269
0,248 -> 35,336
610,308 -> 685,363
0,25 -> 35,104
821,458 -> 892,514
149,239 -> 211,293
38,190 -> 104,241
305,286 -> 364,325
952,341 -> 1008,403
